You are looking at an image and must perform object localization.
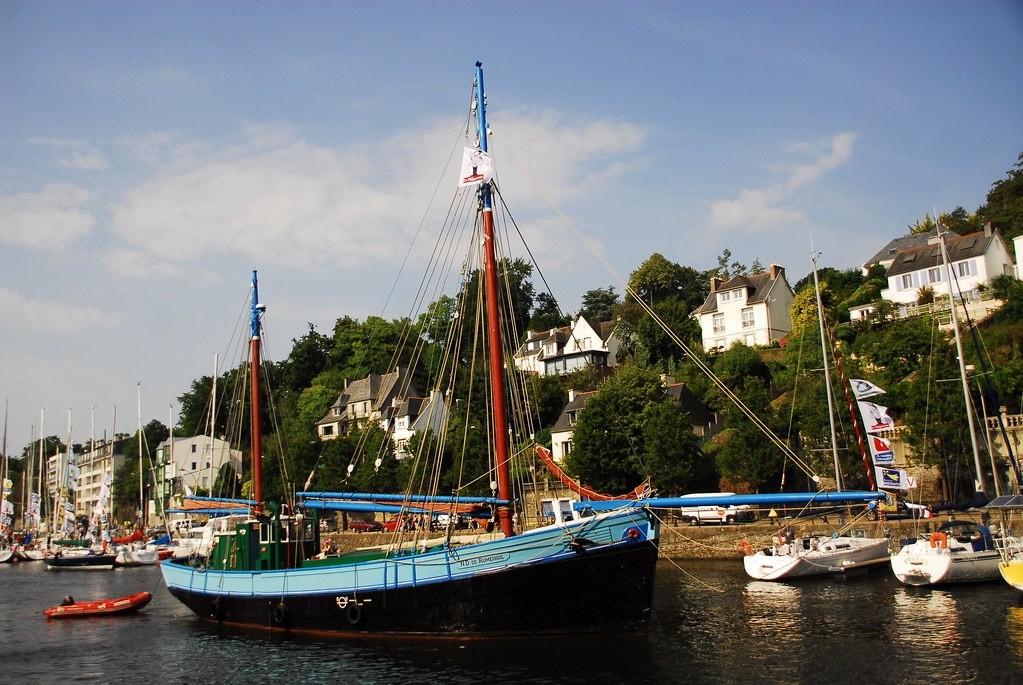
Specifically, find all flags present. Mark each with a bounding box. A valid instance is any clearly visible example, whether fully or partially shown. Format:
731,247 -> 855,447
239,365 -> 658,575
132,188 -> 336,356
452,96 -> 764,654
875,466 -> 908,490
878,490 -> 910,510
1,478 -> 14,526
31,493 -> 41,520
857,400 -> 894,433
459,145 -> 493,187
849,378 -> 886,399
867,434 -> 896,465
85,474 -> 112,553
57,440 -> 79,539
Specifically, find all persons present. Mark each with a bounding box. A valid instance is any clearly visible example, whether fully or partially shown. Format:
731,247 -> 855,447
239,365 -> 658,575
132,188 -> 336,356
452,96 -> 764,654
781,523 -> 798,544
401,513 -> 430,530
317,537 -> 338,559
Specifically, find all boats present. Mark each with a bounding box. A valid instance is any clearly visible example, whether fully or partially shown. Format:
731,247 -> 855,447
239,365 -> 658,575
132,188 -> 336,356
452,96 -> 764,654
43,591 -> 152,617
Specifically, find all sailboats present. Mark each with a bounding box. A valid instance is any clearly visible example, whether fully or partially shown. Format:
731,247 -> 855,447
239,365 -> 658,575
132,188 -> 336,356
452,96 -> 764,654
886,205 -> 1023,594
1,381 -> 179,571
153,63 -> 669,645
737,236 -> 890,582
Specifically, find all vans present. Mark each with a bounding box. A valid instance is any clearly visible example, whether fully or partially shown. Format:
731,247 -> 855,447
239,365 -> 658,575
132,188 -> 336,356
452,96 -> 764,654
432,515 -> 462,531
676,493 -> 752,526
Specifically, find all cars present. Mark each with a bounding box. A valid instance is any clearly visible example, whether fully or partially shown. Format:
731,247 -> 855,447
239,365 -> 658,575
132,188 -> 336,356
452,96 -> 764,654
866,500 -> 934,523
381,514 -> 414,532
349,517 -> 383,533
170,520 -> 198,530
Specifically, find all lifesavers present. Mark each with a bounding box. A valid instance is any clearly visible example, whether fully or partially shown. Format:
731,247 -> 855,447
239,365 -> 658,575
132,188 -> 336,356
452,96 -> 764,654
347,605 -> 362,624
274,608 -> 284,623
930,533 -> 947,549
740,541 -> 752,555
